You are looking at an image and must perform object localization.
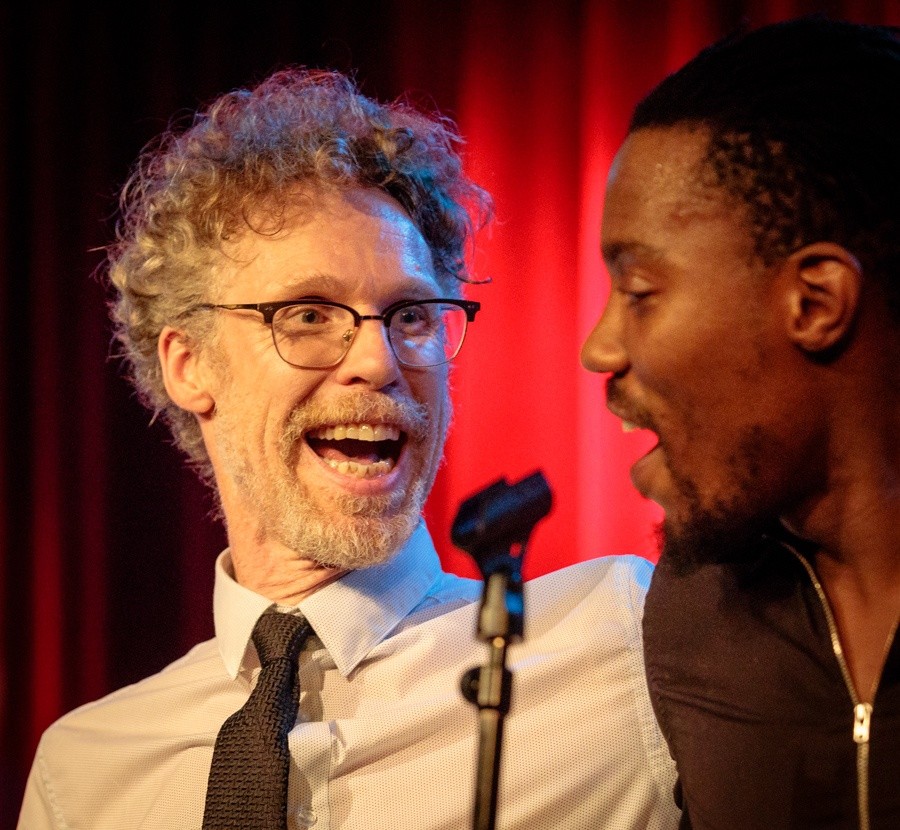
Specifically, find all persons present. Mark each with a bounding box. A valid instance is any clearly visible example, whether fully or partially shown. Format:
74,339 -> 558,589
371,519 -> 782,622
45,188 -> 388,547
583,24 -> 900,830
18,67 -> 682,830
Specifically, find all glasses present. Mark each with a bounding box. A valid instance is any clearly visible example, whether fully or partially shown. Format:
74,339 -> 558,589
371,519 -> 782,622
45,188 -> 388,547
199,299 -> 480,370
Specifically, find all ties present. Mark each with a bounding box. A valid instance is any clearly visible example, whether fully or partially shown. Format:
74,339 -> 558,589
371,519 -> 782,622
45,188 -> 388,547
201,611 -> 316,829
